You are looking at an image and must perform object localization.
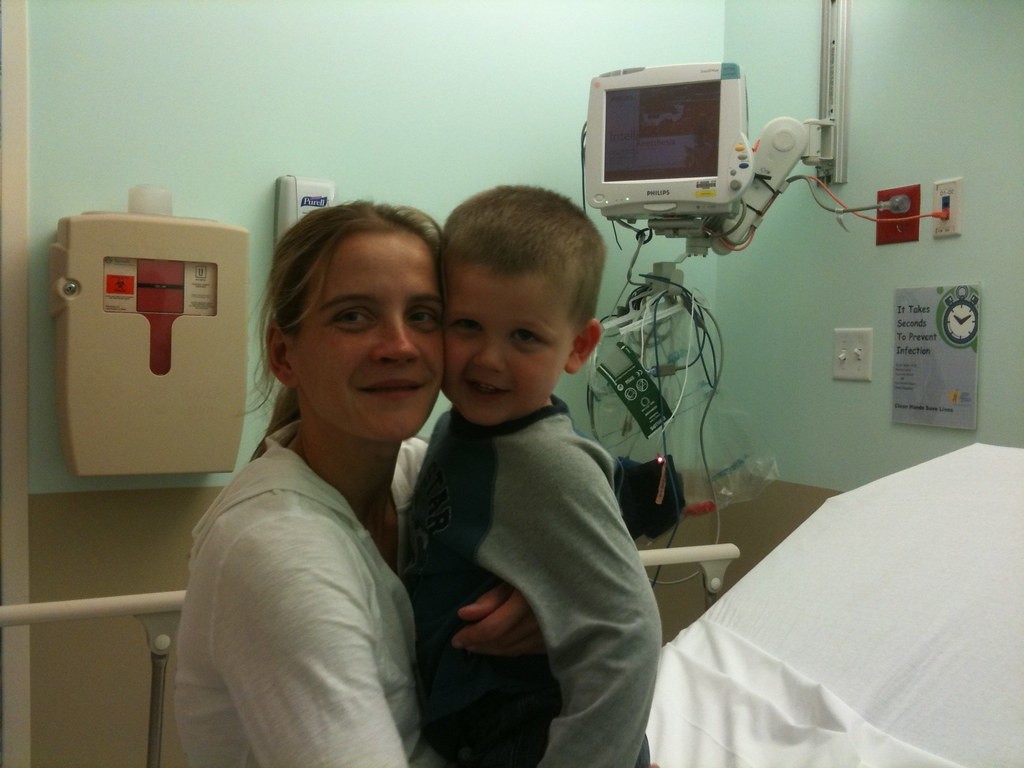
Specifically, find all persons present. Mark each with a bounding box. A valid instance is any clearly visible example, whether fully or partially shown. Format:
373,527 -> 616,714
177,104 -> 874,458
404,185 -> 662,768
173,199 -> 549,768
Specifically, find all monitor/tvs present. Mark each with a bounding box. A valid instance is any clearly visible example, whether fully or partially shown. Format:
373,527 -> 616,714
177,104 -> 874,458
585,62 -> 755,216
